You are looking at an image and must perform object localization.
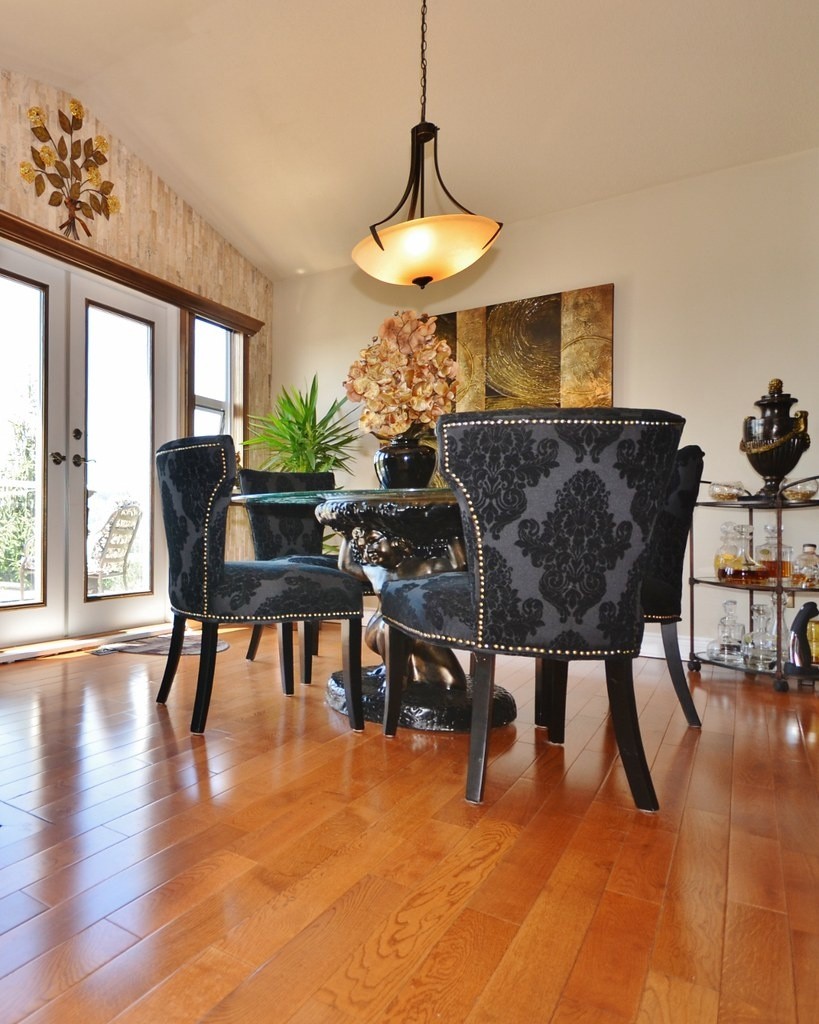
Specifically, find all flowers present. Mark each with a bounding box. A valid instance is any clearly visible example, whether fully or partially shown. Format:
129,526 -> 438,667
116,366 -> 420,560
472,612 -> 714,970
341,308 -> 460,441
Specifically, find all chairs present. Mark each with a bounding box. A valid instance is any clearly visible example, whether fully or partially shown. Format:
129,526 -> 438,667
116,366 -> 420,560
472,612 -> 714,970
378,408 -> 688,812
155,434 -> 365,735
534,445 -> 704,745
20,498 -> 141,603
236,469 -> 375,685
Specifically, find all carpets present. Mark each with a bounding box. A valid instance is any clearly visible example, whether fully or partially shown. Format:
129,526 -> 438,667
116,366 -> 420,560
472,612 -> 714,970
86,629 -> 232,657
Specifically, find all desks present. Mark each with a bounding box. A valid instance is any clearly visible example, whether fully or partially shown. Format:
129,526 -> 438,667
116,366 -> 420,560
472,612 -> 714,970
231,489 -> 517,731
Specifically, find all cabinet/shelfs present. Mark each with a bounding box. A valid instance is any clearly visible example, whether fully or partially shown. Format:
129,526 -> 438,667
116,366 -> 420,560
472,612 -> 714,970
688,475 -> 819,691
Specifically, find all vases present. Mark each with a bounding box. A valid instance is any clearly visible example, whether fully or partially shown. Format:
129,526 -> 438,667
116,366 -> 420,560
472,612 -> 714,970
373,437 -> 438,489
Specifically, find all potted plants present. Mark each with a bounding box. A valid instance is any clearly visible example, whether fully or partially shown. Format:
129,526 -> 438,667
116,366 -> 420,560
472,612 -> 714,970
236,372 -> 365,632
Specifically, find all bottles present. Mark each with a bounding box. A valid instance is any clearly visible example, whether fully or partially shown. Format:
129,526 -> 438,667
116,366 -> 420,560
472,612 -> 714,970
769,591 -> 791,664
740,604 -> 776,671
793,544 -> 819,587
706,623 -> 743,664
717,525 -> 770,586
717,600 -> 745,654
714,521 -> 740,579
755,523 -> 793,582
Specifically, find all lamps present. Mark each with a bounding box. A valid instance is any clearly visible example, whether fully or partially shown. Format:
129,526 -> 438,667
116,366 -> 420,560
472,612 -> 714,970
348,0 -> 503,290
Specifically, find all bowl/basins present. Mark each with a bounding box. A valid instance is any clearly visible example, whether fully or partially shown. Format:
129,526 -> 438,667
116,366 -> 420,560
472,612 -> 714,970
709,481 -> 744,501
779,476 -> 819,501
